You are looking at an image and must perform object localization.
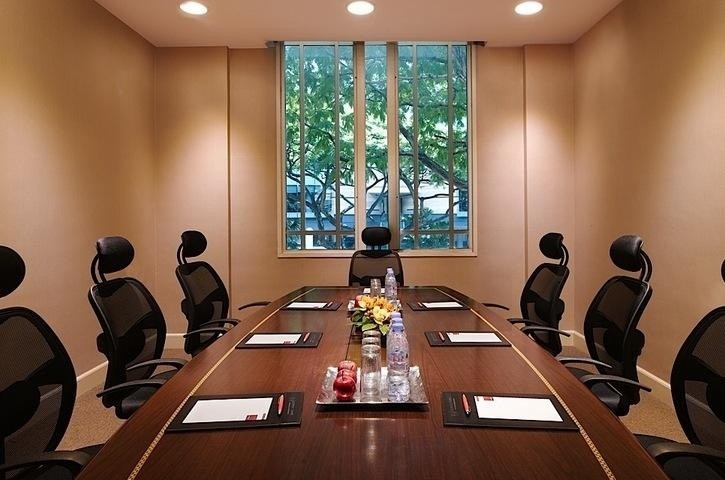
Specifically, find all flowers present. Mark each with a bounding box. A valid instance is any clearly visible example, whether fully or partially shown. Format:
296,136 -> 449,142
348,294 -> 400,336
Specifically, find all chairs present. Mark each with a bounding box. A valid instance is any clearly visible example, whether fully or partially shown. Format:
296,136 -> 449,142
0,245 -> 108,479
348,226 -> 405,287
87,233 -> 189,420
505,232 -> 571,357
631,259 -> 725,480
555,235 -> 656,417
175,229 -> 271,358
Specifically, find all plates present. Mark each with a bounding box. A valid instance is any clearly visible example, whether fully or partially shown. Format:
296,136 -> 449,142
315,365 -> 430,407
346,297 -> 405,313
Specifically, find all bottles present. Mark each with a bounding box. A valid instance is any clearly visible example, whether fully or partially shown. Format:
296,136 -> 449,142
383,266 -> 399,308
385,312 -> 415,404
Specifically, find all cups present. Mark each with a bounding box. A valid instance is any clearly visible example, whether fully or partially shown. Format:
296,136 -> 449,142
369,277 -> 382,303
360,328 -> 382,397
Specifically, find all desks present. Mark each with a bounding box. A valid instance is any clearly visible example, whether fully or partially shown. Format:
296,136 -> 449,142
76,284 -> 669,480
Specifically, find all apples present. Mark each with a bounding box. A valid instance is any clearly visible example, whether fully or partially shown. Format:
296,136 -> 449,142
354,295 -> 364,307
334,358 -> 357,401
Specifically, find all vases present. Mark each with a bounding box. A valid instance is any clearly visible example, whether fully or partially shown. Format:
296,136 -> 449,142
354,326 -> 387,348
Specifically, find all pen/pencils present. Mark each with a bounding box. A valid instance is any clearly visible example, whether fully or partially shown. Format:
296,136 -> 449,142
417,302 -> 423,307
277,394 -> 284,415
327,301 -> 333,307
438,331 -> 445,341
462,393 -> 472,415
303,332 -> 310,342
